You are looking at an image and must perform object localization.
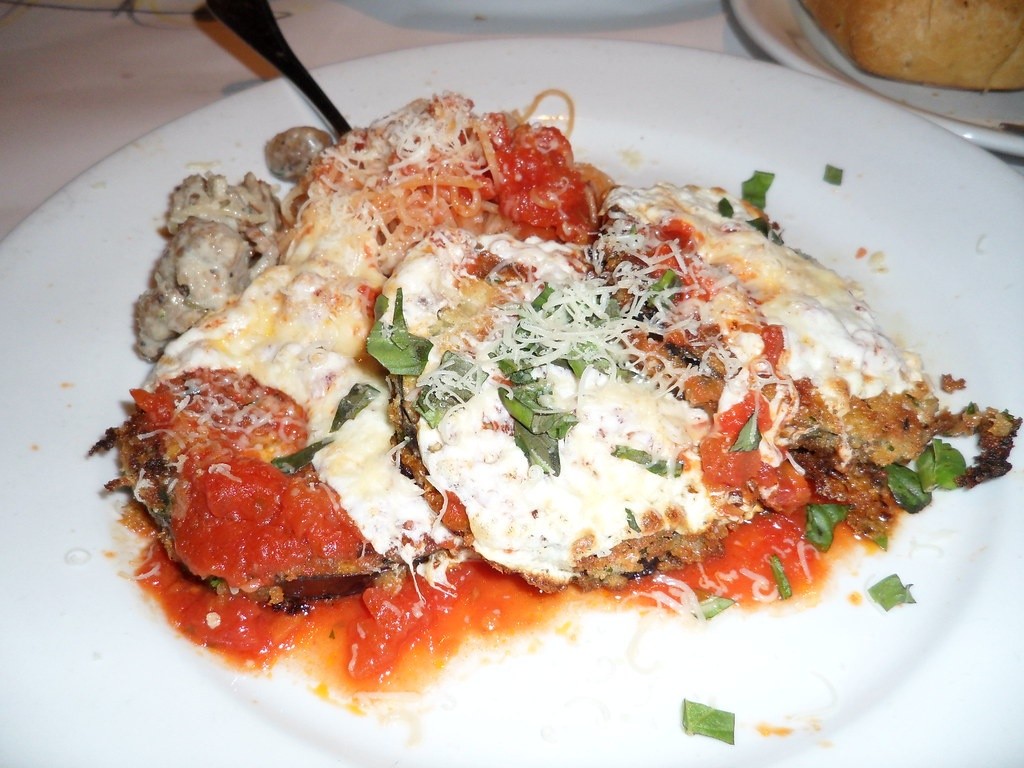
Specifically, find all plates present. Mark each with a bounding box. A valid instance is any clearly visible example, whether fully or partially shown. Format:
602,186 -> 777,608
724,1 -> 1024,164
3,33 -> 1024,765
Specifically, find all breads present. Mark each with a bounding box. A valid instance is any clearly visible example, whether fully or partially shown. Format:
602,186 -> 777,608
794,0 -> 1024,92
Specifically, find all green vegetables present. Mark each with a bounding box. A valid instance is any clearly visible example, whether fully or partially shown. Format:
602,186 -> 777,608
272,164 -> 967,746
